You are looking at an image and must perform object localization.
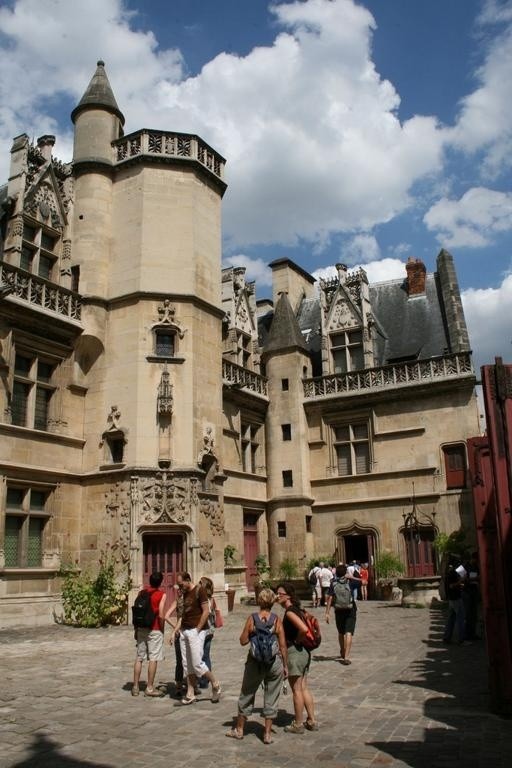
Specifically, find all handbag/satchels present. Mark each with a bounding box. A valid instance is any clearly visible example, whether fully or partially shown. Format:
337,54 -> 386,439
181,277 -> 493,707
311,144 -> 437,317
213,597 -> 223,628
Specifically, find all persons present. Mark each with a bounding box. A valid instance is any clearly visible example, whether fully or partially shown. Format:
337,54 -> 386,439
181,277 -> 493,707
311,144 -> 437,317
360,563 -> 368,601
308,561 -> 323,607
276,583 -> 320,735
324,565 -> 368,666
322,563 -> 334,606
198,577 -> 219,688
225,588 -> 288,745
464,552 -> 480,641
443,569 -> 467,643
353,560 -> 360,569
320,561 -> 324,568
131,572 -> 167,697
164,593 -> 201,695
347,562 -> 359,602
169,572 -> 221,707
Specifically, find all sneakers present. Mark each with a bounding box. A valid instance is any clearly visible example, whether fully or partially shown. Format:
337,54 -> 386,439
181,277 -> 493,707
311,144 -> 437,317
303,720 -> 319,731
132,688 -> 139,696
285,720 -> 304,733
211,681 -> 222,702
226,728 -> 243,739
344,659 -> 351,665
145,687 -> 165,697
264,732 -> 273,744
175,695 -> 195,706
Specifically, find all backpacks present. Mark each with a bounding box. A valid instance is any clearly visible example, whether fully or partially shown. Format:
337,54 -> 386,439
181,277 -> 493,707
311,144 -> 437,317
250,612 -> 279,663
131,589 -> 157,629
332,579 -> 353,608
309,569 -> 321,585
298,609 -> 322,650
